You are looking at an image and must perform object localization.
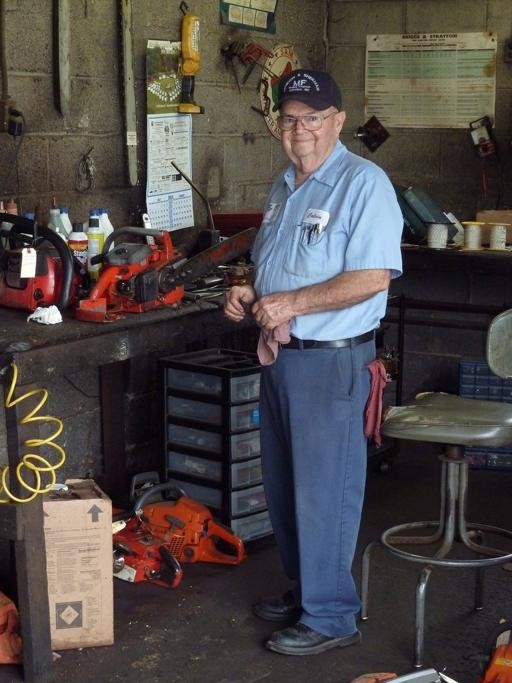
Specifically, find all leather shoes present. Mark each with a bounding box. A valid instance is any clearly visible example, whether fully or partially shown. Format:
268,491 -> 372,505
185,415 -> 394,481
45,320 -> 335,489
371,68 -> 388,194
266,620 -> 362,656
252,590 -> 302,621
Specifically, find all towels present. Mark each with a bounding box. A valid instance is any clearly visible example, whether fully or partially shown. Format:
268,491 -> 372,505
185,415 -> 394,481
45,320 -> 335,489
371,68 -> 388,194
256,319 -> 292,365
364,358 -> 390,448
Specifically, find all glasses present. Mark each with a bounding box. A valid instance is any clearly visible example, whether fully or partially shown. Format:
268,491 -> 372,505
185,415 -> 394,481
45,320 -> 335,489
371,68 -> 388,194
277,112 -> 336,131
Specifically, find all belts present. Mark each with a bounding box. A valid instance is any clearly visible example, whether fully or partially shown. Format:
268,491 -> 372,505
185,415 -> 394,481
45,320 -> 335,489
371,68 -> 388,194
281,330 -> 375,349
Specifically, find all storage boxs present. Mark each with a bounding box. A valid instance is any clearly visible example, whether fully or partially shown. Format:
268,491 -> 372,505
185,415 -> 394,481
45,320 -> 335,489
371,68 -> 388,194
17,480 -> 116,650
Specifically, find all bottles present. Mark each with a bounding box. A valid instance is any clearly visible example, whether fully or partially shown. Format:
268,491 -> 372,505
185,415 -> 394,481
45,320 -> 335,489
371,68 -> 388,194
0,198 -> 114,280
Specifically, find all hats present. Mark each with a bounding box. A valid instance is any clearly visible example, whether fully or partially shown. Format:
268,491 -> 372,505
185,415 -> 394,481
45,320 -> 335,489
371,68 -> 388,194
272,69 -> 341,113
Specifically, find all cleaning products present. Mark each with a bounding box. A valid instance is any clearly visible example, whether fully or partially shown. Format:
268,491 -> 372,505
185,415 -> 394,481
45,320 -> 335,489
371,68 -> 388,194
45,208 -> 115,282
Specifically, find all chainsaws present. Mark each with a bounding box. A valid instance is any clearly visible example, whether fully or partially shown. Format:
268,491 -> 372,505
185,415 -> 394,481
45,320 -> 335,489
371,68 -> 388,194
83,225 -> 258,314
111,473 -> 245,589
0,211 -> 87,311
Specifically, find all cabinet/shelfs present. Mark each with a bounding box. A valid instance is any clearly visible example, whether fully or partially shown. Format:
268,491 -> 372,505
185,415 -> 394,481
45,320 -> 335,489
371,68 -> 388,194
158,346 -> 274,545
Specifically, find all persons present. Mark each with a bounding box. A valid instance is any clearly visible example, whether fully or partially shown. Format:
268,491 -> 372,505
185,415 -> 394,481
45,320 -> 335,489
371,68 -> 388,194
223,70 -> 404,656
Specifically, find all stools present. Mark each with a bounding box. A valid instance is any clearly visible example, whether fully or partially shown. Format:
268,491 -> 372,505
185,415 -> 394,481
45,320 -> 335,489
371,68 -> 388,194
360,388 -> 511,665
